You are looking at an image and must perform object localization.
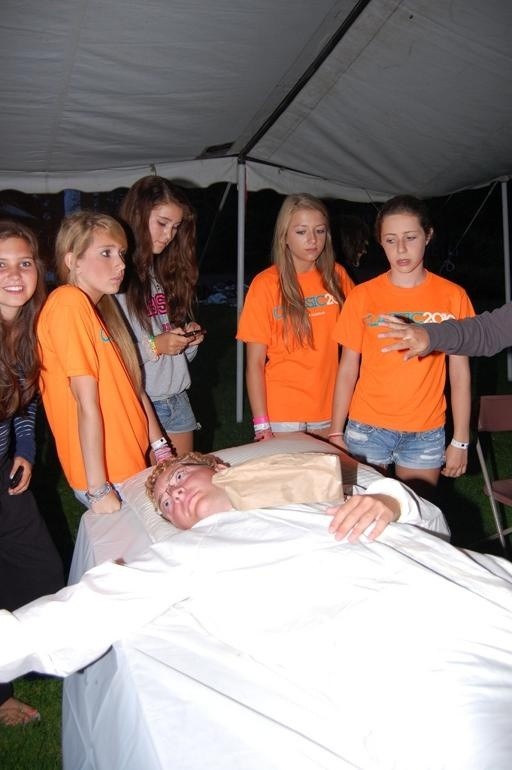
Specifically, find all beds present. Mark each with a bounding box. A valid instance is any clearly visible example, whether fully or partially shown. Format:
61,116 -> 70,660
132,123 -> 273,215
81,491 -> 511,770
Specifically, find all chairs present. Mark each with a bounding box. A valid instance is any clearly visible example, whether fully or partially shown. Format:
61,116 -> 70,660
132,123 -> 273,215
470,394 -> 512,551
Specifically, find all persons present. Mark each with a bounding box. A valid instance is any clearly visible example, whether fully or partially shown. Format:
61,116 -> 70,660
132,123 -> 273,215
35,214 -> 175,509
378,305 -> 512,362
0,453 -> 452,686
331,198 -> 475,499
0,218 -> 67,727
118,176 -> 206,455
234,193 -> 354,438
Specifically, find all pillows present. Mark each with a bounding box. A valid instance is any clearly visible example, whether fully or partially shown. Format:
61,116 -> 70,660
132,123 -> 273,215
116,429 -> 390,547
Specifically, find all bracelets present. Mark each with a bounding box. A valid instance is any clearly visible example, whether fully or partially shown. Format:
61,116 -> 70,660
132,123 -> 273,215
449,439 -> 469,450
149,436 -> 167,450
85,481 -> 113,505
327,433 -> 344,438
149,336 -> 161,356
250,416 -> 271,431
153,448 -> 172,462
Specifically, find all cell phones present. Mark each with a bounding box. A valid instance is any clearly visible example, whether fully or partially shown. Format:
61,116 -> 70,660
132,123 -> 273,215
185,331 -> 198,337
